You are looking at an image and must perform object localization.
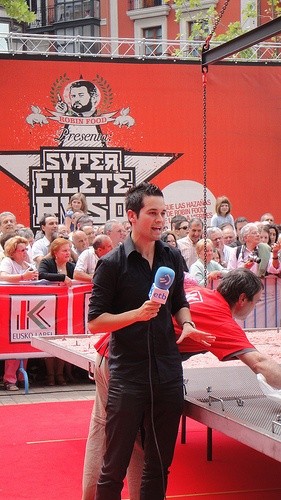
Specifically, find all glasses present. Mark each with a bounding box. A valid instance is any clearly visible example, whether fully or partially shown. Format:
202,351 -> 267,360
192,229 -> 202,232
179,226 -> 187,230
247,231 -> 261,235
167,240 -> 175,243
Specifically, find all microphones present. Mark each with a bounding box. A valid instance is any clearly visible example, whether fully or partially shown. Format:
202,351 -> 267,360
148,266 -> 175,305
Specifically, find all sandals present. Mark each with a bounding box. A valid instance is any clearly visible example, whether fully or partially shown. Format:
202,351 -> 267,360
17,381 -> 31,388
5,382 -> 19,391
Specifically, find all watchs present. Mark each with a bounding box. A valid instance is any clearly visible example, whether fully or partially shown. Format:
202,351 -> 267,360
183,321 -> 196,328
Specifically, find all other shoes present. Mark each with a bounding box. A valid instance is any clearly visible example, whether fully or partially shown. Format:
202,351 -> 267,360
48,374 -> 55,386
56,373 -> 67,386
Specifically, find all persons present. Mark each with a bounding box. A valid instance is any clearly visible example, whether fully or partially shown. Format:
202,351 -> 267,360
160,195 -> 281,288
81,268 -> 281,500
0,193 -> 131,390
86,180 -> 217,500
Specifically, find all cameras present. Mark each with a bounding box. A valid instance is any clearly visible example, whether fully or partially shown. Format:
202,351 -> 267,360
248,253 -> 261,264
